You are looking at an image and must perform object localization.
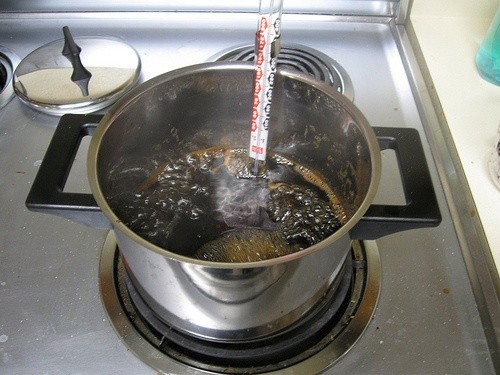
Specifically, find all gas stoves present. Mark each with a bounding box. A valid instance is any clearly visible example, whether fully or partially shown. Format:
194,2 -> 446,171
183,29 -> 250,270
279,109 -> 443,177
1,8 -> 500,375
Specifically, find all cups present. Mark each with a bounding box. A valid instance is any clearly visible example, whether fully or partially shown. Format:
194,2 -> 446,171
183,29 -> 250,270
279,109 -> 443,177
476,6 -> 500,85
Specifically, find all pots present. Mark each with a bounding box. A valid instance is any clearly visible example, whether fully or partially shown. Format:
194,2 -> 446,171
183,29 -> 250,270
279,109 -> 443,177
26,62 -> 445,335
